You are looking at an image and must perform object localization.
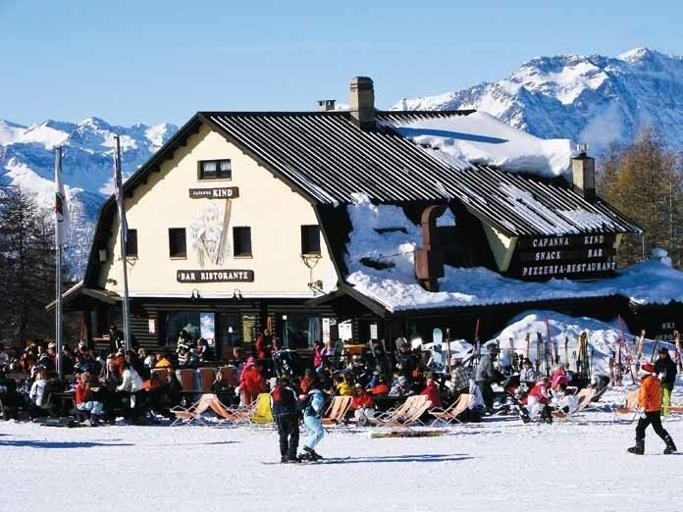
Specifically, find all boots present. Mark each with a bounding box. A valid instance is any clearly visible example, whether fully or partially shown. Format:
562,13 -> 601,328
627,431 -> 677,456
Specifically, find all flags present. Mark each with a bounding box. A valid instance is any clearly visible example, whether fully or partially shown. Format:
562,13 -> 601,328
110,153 -> 129,245
54,160 -> 71,248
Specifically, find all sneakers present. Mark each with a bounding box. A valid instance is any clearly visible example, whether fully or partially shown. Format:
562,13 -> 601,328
280,447 -> 322,464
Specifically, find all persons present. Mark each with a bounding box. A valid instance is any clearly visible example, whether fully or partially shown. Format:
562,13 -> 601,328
625,362 -> 678,455
0,322 -> 680,427
297,380 -> 325,461
268,379 -> 304,464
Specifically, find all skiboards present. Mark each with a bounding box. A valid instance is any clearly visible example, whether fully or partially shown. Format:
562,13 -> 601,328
259,452 -> 350,464
469,338 -> 481,368
369,338 -> 393,373
371,430 -> 449,438
509,329 -> 683,387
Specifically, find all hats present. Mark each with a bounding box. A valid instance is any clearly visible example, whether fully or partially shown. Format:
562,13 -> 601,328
640,348 -> 669,375
450,358 -> 459,366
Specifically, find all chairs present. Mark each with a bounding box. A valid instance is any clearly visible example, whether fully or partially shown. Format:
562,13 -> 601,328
197,367 -> 219,392
0,378 -> 116,426
150,367 -> 173,384
217,366 -> 235,385
168,385 -> 683,437
175,368 -> 195,390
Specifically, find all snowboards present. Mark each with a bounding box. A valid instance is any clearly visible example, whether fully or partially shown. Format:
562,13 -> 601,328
433,329 -> 444,372
